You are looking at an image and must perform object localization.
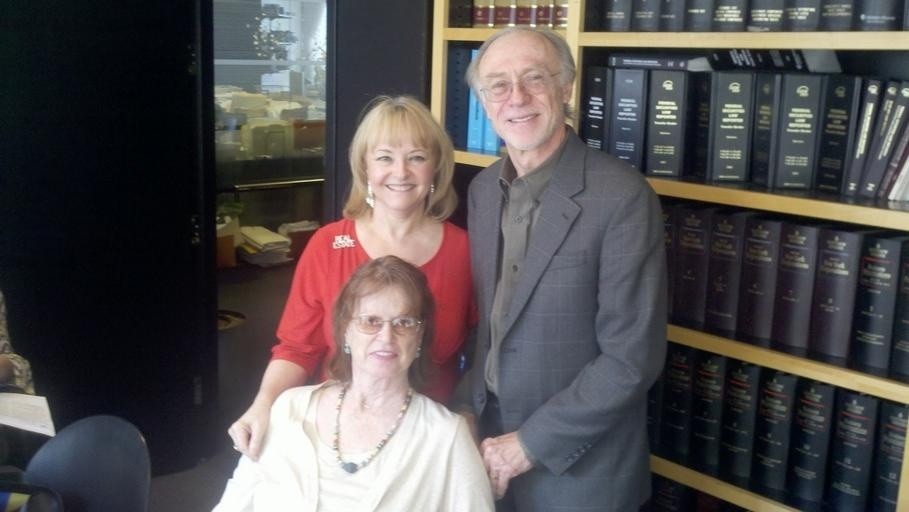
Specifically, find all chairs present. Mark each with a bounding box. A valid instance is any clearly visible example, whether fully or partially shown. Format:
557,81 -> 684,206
21,415 -> 152,512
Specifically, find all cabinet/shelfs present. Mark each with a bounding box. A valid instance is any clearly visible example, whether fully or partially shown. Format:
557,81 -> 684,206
430,0 -> 909,512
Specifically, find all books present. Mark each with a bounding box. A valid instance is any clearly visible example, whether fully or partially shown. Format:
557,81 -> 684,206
215,83 -> 321,270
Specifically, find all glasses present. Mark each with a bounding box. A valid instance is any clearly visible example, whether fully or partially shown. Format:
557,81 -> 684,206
349,312 -> 426,336
478,69 -> 561,103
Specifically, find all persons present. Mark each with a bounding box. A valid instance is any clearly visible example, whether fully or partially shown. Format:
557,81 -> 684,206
209,254 -> 496,511
227,96 -> 479,463
0,292 -> 36,466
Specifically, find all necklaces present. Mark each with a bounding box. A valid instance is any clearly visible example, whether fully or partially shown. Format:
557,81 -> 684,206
331,383 -> 413,472
